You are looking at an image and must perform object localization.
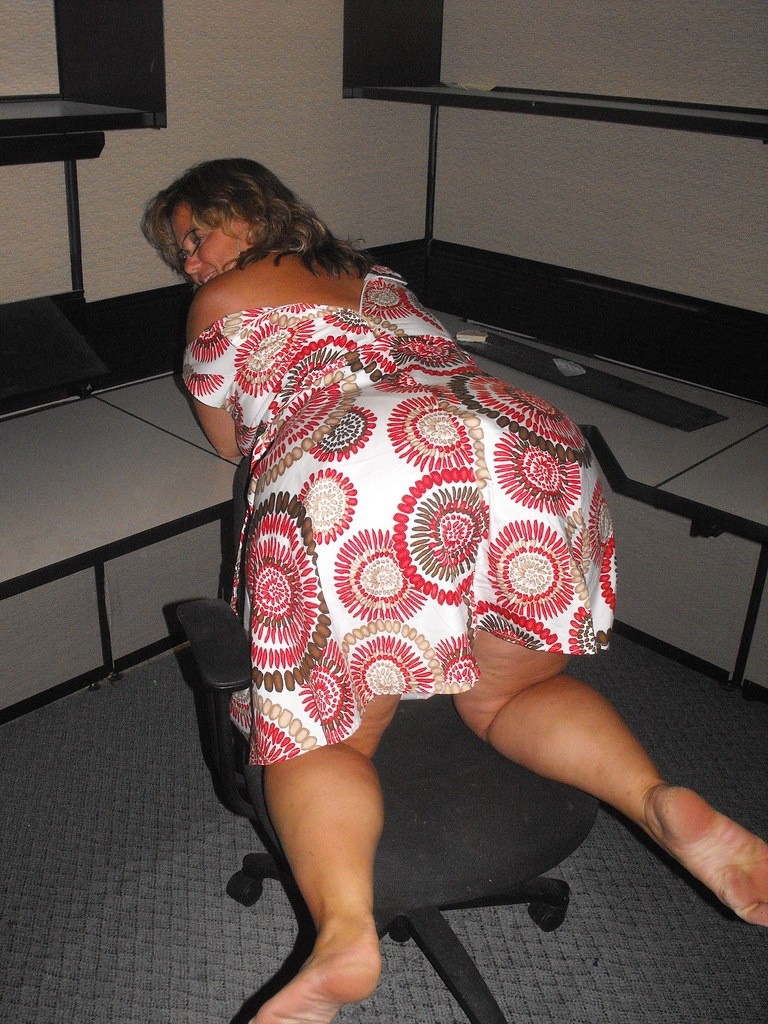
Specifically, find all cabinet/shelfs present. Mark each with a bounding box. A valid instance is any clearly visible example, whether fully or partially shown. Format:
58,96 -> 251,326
0,309 -> 768,730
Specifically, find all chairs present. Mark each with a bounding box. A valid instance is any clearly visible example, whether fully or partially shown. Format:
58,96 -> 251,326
176,459 -> 599,1024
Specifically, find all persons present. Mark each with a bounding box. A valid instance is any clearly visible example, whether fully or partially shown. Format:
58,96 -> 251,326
166,154 -> 768,1024
